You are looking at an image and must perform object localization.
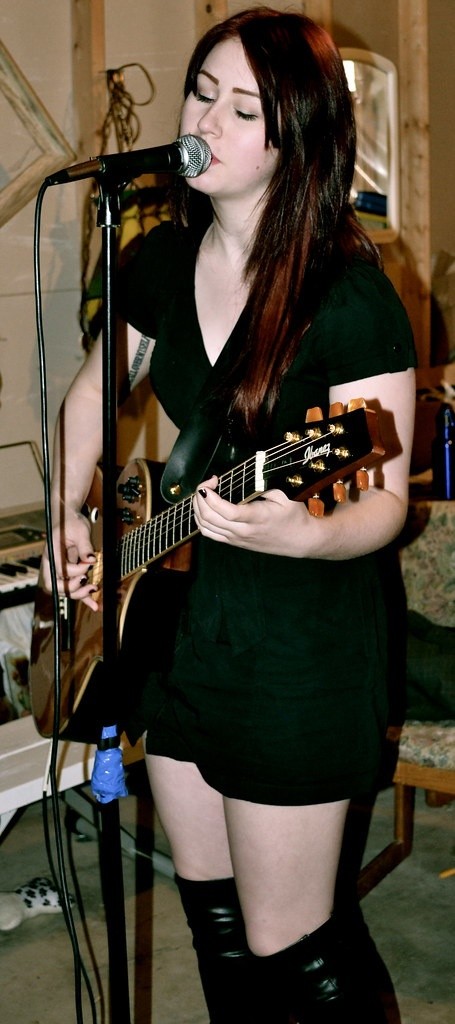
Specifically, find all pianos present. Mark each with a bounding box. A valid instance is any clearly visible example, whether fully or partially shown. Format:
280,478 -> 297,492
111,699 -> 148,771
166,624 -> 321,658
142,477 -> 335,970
0,521 -> 151,844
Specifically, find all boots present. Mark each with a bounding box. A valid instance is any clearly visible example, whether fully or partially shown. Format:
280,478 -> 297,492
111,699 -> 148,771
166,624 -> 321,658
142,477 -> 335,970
252,904 -> 402,1024
174,875 -> 252,1024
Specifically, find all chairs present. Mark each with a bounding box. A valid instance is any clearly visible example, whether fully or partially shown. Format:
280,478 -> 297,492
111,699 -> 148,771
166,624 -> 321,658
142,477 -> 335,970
391,502 -> 455,839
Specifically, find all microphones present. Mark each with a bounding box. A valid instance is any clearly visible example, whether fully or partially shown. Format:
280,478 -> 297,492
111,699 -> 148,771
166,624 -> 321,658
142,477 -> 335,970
43,134 -> 212,186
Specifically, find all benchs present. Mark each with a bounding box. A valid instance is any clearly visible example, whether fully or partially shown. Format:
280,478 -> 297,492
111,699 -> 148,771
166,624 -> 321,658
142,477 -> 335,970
0,714 -> 177,885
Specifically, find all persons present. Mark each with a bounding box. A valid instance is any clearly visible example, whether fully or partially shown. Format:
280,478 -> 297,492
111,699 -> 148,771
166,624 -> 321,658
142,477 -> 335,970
41,6 -> 416,1024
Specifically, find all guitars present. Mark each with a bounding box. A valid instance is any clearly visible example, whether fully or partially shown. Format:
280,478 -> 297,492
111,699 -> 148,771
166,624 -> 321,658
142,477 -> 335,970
25,400 -> 393,743
